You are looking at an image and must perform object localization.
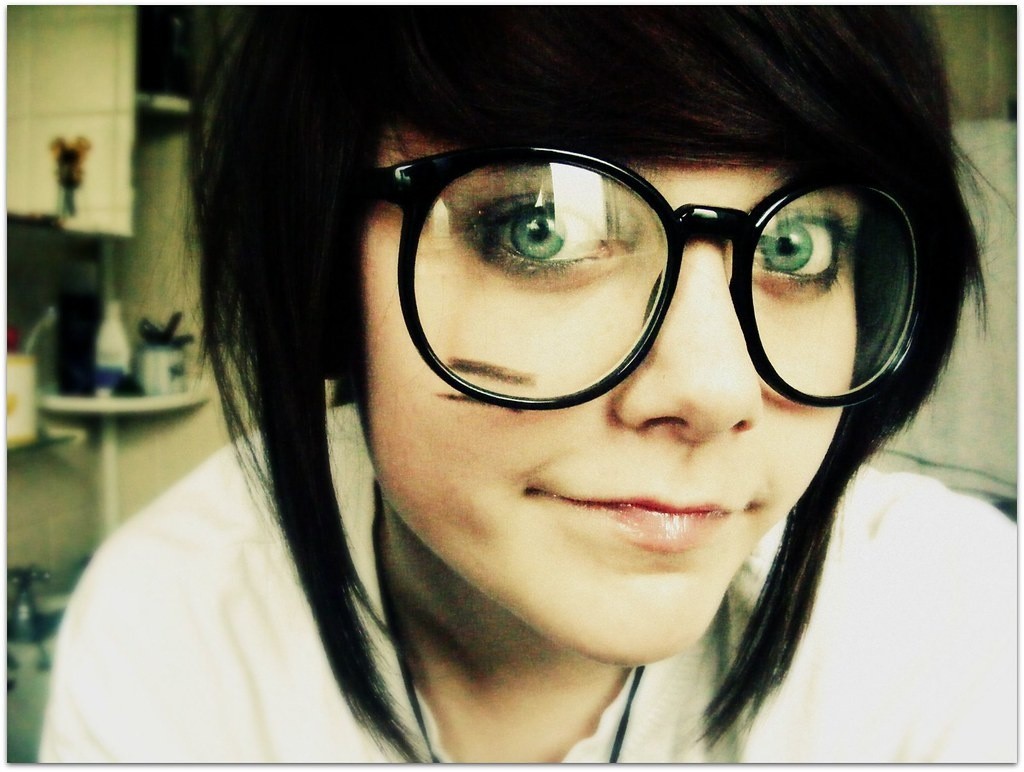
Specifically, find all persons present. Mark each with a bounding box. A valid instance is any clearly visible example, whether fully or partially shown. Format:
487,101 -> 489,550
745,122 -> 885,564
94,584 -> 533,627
41,5 -> 1017,763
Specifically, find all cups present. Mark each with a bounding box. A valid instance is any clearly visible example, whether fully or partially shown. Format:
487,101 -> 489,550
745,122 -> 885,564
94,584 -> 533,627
141,343 -> 185,391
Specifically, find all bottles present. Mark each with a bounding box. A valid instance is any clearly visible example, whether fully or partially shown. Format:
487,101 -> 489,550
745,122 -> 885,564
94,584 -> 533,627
94,300 -> 130,397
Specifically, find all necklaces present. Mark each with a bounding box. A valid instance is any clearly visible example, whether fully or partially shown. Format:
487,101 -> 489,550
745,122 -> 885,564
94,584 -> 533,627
368,481 -> 647,765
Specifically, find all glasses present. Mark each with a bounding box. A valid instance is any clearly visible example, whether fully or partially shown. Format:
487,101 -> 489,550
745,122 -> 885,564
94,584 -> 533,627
364,141 -> 922,412
